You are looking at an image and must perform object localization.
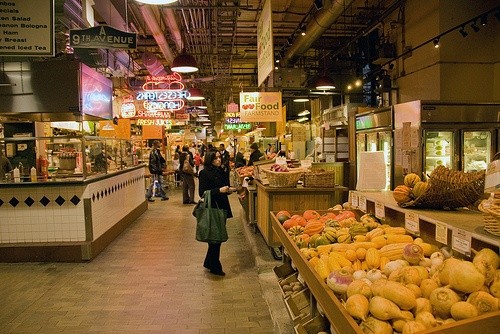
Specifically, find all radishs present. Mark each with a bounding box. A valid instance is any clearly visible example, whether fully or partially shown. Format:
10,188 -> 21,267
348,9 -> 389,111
327,243 -> 454,296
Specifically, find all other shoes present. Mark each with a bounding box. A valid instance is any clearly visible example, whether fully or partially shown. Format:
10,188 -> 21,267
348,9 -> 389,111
161,196 -> 169,200
148,197 -> 155,201
192,200 -> 198,204
183,199 -> 192,204
214,271 -> 225,275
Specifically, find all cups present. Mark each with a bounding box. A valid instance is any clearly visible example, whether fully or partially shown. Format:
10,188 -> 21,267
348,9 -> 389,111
86,163 -> 91,174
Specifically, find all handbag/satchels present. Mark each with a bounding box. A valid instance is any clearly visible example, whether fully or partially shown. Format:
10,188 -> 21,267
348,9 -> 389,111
182,154 -> 194,175
191,190 -> 228,244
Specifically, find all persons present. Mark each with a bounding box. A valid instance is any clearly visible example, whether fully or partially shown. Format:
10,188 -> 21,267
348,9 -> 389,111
0,145 -> 12,179
145,140 -> 169,202
219,144 -> 232,187
270,144 -> 276,152
198,143 -> 217,156
199,148 -> 236,276
172,152 -> 181,186
176,145 -> 181,152
265,144 -> 271,153
98,149 -> 113,163
183,142 -> 201,204
248,143 -> 263,166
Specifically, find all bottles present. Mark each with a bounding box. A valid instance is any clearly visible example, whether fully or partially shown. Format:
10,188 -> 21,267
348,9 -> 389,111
31,166 -> 36,180
14,167 -> 20,177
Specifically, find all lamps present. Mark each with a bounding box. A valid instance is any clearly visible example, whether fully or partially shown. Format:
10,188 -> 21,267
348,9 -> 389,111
389,62 -> 394,71
494,6 -> 500,21
316,47 -> 338,91
185,84 -> 211,131
459,25 -> 469,39
171,26 -> 199,73
482,15 -> 489,25
471,20 -> 480,32
433,38 -> 441,48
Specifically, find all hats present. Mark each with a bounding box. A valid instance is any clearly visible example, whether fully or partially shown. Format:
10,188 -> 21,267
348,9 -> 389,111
250,143 -> 259,149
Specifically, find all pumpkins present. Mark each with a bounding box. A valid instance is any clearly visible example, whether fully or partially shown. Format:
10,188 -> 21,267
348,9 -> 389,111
392,173 -> 429,204
276,209 -> 384,258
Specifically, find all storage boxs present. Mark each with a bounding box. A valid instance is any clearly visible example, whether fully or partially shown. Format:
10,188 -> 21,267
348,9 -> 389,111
274,203 -> 500,333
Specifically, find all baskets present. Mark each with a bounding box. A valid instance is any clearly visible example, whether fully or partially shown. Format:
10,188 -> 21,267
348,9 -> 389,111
303,170 -> 335,187
478,193 -> 500,238
263,167 -> 305,187
